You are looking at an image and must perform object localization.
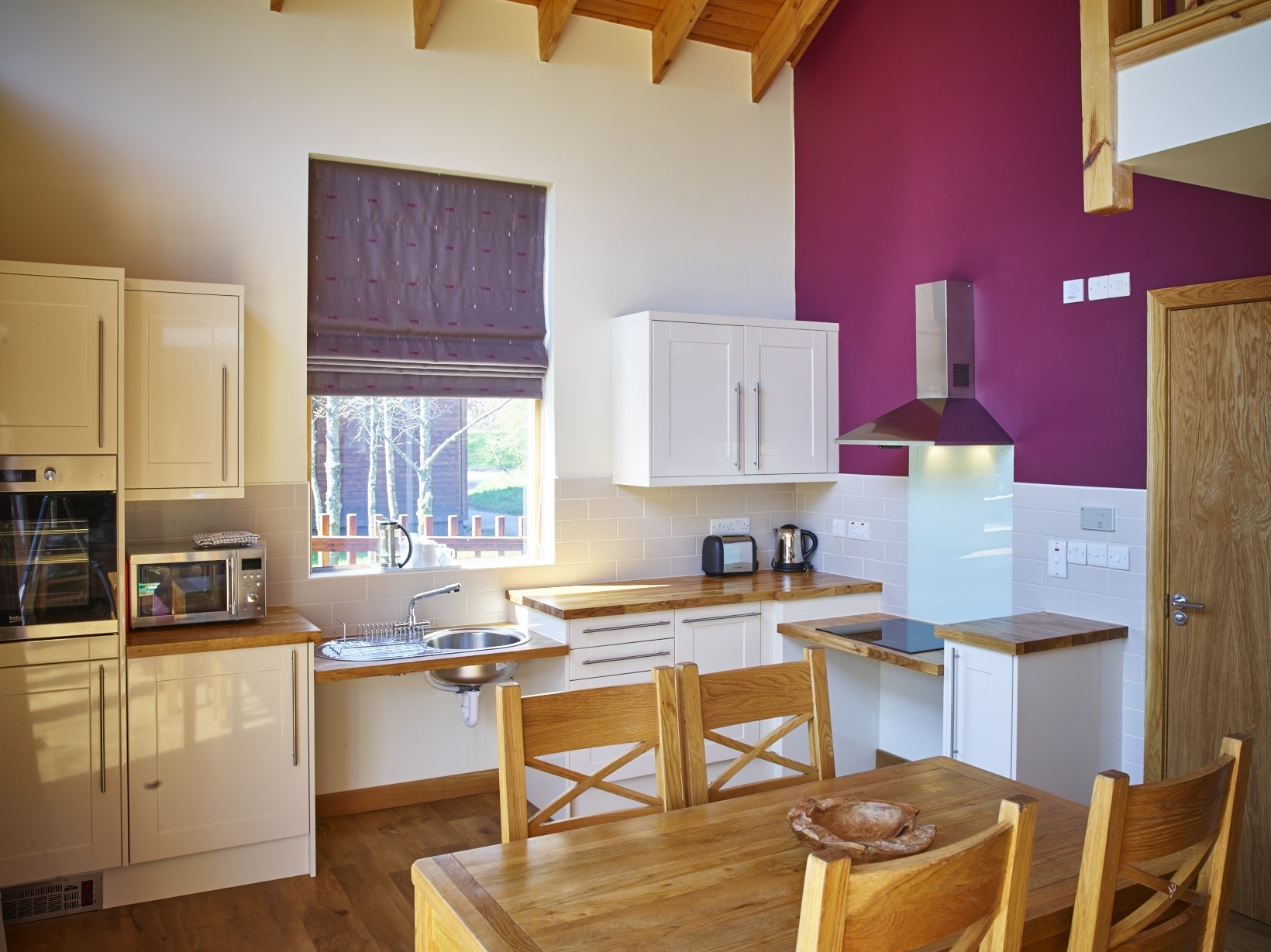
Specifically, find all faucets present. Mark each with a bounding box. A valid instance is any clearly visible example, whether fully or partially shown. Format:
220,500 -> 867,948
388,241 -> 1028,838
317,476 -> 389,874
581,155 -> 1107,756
392,583 -> 462,641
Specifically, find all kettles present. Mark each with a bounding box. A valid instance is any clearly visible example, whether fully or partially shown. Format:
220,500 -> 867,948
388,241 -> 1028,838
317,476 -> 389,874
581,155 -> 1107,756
771,524 -> 817,572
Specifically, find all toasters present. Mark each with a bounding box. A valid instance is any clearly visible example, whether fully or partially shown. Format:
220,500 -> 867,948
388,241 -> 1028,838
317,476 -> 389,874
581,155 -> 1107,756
702,534 -> 759,577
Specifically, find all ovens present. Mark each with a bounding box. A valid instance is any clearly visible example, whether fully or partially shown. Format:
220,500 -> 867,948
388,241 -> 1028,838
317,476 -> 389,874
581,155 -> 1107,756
0,455 -> 120,643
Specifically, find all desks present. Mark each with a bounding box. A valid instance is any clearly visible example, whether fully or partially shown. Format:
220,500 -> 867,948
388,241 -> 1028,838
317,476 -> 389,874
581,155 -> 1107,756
777,612 -> 944,676
411,752 -> 1207,952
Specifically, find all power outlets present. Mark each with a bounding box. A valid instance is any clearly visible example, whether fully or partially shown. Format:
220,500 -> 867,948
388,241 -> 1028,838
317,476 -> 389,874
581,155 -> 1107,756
711,517 -> 750,536
849,521 -> 869,541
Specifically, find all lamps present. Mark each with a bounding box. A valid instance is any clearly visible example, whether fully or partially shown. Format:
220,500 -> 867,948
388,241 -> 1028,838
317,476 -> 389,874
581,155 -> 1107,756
834,280 -> 1015,446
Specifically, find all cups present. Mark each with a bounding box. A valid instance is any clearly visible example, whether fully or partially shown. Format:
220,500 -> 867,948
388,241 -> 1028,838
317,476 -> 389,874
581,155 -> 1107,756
401,534 -> 437,567
437,544 -> 455,566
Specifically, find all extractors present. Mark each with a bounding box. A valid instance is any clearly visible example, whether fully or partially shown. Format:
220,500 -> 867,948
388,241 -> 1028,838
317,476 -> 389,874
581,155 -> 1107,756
833,280 -> 1015,446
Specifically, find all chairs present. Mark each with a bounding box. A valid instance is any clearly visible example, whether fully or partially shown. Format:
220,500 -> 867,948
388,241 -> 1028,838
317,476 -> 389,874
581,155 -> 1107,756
794,794 -> 1039,952
497,648 -> 836,844
1067,733 -> 1253,952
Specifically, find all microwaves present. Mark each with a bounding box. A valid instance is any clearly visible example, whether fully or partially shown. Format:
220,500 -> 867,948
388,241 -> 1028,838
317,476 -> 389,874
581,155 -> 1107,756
126,543 -> 267,628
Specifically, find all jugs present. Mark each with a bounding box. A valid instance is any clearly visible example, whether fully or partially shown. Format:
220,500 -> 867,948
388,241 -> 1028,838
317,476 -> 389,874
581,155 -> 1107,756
377,520 -> 413,568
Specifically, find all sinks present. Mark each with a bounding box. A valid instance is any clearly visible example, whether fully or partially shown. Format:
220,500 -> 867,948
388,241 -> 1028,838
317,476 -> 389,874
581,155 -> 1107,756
421,628 -> 530,685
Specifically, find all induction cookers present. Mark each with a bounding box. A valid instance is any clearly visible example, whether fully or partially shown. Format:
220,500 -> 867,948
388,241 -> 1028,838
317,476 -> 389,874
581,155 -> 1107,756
815,618 -> 944,654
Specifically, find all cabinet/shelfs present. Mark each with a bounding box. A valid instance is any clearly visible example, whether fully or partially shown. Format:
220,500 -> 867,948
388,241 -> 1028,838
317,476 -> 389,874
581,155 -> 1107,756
0,261 -> 125,454
934,610 -> 1128,807
126,642 -> 317,897
509,602 -> 774,820
0,632 -> 123,887
610,311 -> 840,487
125,278 -> 245,501
